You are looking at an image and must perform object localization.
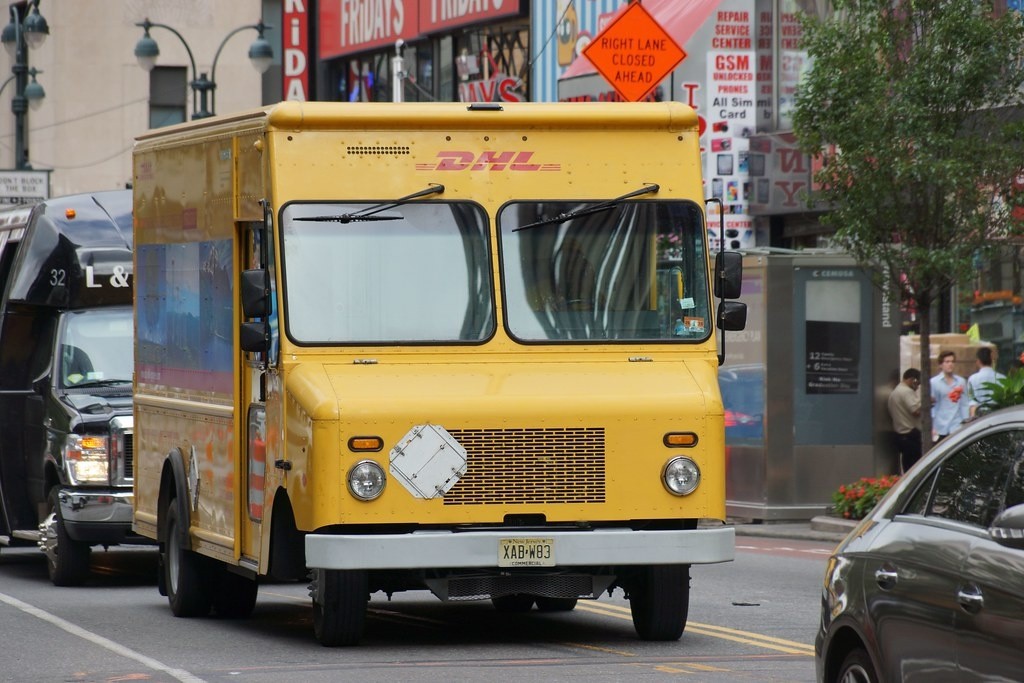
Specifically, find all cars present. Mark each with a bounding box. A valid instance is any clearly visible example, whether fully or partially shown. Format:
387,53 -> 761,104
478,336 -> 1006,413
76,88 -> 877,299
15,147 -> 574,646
814,404 -> 1024,683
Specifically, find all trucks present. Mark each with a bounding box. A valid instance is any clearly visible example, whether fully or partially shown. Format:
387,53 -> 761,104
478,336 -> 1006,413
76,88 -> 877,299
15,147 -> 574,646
130,97 -> 748,649
0,189 -> 164,589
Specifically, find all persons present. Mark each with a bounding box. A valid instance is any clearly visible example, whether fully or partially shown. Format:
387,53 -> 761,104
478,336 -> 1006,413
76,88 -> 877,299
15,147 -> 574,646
930,351 -> 977,446
527,238 -> 614,312
888,368 -> 937,473
968,347 -> 1006,411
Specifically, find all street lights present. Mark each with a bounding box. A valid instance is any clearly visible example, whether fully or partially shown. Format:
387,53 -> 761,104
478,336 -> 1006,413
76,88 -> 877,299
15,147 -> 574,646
134,16 -> 272,121
0,0 -> 49,169
